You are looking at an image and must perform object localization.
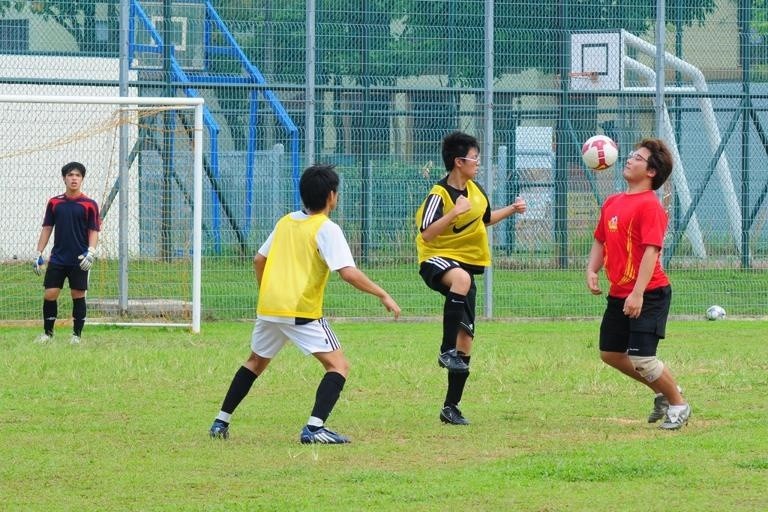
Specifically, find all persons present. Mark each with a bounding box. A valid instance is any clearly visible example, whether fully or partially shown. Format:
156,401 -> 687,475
587,139 -> 692,429
415,131 -> 526,426
209,162 -> 401,443
32,163 -> 101,345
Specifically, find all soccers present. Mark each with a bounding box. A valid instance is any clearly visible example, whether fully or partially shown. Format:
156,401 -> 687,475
581,135 -> 617,169
705,306 -> 725,321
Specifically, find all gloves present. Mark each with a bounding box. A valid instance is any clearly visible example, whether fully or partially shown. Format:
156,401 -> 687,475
31,250 -> 45,277
77,246 -> 98,272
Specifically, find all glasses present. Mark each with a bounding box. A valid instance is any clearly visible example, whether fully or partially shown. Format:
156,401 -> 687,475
457,153 -> 483,163
628,150 -> 649,165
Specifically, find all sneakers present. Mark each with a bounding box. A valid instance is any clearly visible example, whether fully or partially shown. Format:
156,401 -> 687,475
33,333 -> 53,345
209,421 -> 230,438
438,346 -> 470,375
300,424 -> 352,444
648,386 -> 682,423
439,405 -> 473,425
656,400 -> 691,431
69,334 -> 81,345
